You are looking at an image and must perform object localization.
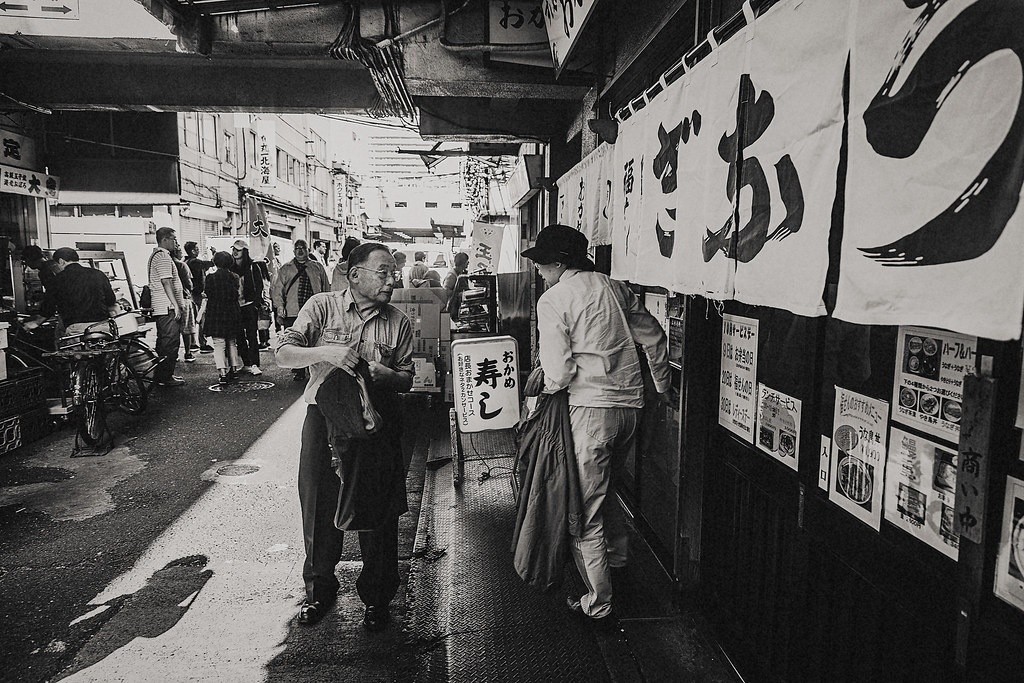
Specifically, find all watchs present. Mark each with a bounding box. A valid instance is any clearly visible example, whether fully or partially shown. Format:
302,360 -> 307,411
204,252 -> 237,392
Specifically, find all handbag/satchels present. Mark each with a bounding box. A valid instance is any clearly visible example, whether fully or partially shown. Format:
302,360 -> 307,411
140,285 -> 152,308
272,297 -> 287,311
257,303 -> 273,330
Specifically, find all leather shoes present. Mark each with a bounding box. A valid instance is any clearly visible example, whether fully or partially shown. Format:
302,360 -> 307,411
364,603 -> 387,628
297,590 -> 337,623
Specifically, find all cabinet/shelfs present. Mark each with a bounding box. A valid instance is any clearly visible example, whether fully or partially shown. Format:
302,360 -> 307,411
455,274 -> 497,336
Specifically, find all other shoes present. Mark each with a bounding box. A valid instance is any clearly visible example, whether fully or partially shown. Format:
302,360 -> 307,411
258,343 -> 268,352
242,365 -> 262,376
189,344 -> 200,352
217,374 -> 227,384
153,374 -> 183,385
265,343 -> 272,348
184,353 -> 196,362
200,345 -> 214,353
293,372 -> 306,381
610,566 -> 626,582
68,407 -> 87,430
566,595 -> 615,630
228,371 -> 239,379
105,398 -> 121,413
158,376 -> 185,386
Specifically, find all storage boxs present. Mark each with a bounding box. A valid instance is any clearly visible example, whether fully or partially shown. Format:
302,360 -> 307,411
0,322 -> 51,455
391,288 -> 453,403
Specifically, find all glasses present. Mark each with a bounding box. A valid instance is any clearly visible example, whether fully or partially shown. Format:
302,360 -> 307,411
356,266 -> 401,282
293,246 -> 308,250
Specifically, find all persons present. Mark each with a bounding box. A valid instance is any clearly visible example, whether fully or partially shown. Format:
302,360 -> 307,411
231,240 -> 281,375
521,225 -> 673,622
332,237 -> 360,291
391,252 -> 469,297
20,244 -> 117,388
273,242 -> 415,629
147,228 -> 217,386
312,241 -> 326,266
274,239 -> 330,381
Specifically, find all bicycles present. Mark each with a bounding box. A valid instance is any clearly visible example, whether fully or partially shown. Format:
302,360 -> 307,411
1,306 -> 172,458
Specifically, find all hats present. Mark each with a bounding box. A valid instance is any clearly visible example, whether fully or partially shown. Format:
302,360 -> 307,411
230,239 -> 249,250
520,224 -> 596,273
